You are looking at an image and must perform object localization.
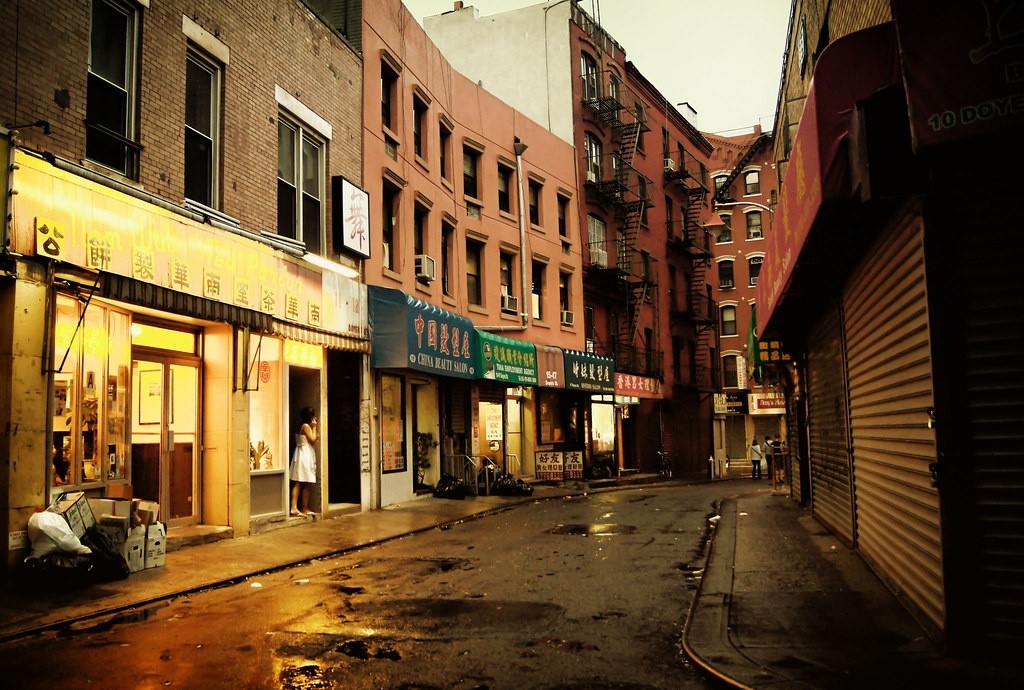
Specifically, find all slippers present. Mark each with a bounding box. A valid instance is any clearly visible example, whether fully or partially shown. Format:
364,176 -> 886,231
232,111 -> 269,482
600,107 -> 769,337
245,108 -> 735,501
308,511 -> 314,514
291,513 -> 307,518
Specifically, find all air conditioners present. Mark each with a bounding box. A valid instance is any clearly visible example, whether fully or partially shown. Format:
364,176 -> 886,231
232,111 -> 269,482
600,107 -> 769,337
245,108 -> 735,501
664,157 -> 675,171
562,311 -> 574,327
751,231 -> 761,238
501,296 -> 519,312
415,254 -> 436,283
751,277 -> 759,285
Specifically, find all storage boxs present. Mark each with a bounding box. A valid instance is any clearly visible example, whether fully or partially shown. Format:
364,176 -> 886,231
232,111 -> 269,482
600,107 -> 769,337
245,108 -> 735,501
46,490 -> 167,572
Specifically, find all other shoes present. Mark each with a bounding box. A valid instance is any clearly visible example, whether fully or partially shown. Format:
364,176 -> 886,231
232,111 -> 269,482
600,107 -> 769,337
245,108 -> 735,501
776,479 -> 786,485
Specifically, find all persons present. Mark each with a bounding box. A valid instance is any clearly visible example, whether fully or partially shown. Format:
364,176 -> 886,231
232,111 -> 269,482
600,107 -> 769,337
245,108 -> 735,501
289,407 -> 317,515
762,432 -> 786,484
751,439 -> 761,480
